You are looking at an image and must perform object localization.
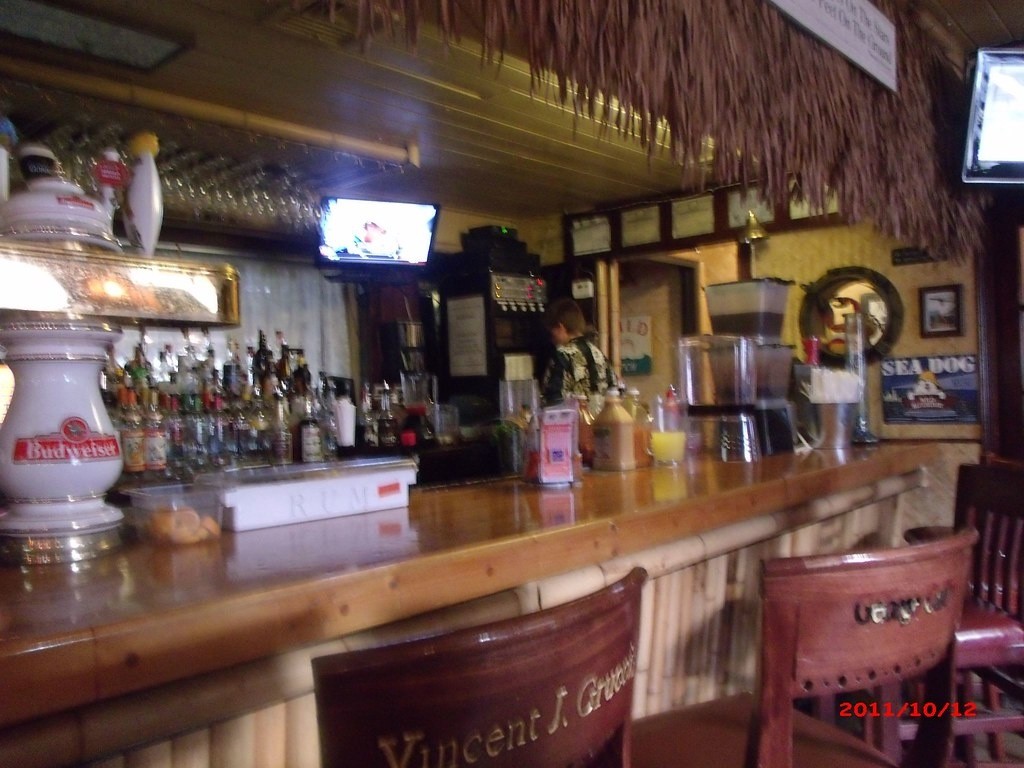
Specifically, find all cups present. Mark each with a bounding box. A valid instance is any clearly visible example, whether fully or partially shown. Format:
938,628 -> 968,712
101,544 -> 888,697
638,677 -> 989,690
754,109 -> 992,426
644,398 -> 689,470
498,380 -> 539,475
807,402 -> 856,451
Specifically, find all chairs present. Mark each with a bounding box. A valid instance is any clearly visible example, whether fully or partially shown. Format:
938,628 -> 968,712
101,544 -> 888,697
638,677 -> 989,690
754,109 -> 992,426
634,525 -> 980,768
310,565 -> 648,768
833,461 -> 1024,768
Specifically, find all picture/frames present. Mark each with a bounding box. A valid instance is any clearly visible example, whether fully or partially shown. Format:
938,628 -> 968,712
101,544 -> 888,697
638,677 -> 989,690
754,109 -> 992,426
917,283 -> 965,340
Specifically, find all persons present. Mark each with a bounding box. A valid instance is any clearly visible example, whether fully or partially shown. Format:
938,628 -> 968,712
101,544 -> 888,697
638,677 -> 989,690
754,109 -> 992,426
535,298 -> 627,407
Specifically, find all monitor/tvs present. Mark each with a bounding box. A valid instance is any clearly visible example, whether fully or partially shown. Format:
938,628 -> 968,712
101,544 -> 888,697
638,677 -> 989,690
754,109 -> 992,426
961,46 -> 1024,188
311,195 -> 440,282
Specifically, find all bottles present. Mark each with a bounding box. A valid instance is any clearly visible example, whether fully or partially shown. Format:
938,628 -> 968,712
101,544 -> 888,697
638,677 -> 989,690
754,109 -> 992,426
100,326 -> 458,490
791,336 -> 828,447
565,383 -> 689,472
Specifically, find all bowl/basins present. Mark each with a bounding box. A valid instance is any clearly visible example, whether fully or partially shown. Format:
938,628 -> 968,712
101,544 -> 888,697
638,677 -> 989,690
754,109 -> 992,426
117,482 -> 230,546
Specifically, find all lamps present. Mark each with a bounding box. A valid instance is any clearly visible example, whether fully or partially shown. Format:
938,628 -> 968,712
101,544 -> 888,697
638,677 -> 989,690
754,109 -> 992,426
735,209 -> 770,245
